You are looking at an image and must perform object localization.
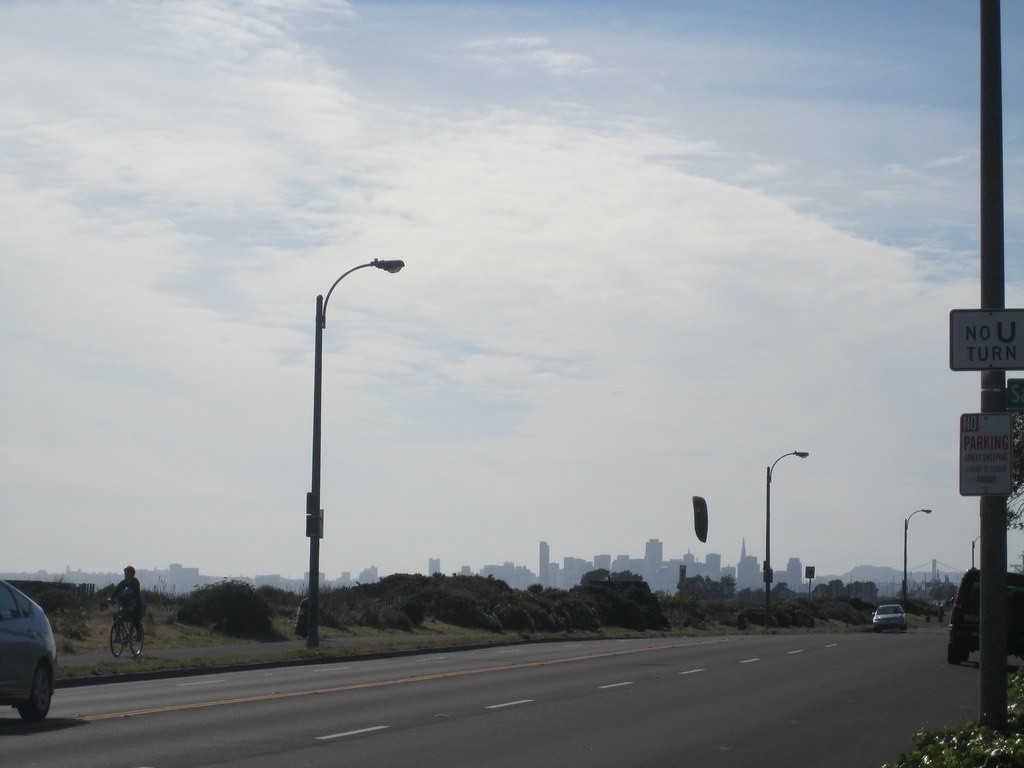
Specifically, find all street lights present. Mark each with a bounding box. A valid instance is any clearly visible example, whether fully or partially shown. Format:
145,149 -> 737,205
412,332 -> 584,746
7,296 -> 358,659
306,259 -> 405,646
904,509 -> 932,610
763,451 -> 810,629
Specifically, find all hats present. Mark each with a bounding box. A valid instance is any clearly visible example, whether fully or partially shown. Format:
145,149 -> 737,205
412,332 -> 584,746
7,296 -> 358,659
124,566 -> 135,576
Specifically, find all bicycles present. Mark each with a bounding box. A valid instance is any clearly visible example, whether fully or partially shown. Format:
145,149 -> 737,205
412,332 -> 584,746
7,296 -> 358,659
108,597 -> 145,656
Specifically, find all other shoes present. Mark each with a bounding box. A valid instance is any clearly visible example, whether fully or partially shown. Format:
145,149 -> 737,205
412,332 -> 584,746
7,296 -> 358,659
113,639 -> 121,643
137,631 -> 142,640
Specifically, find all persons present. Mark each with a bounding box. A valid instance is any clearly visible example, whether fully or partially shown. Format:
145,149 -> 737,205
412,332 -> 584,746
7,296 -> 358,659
107,566 -> 144,641
926,604 -> 931,622
938,604 -> 945,622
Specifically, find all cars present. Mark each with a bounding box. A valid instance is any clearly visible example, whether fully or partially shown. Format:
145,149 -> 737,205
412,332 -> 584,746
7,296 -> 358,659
0,578 -> 58,719
948,567 -> 1024,665
873,606 -> 907,632
